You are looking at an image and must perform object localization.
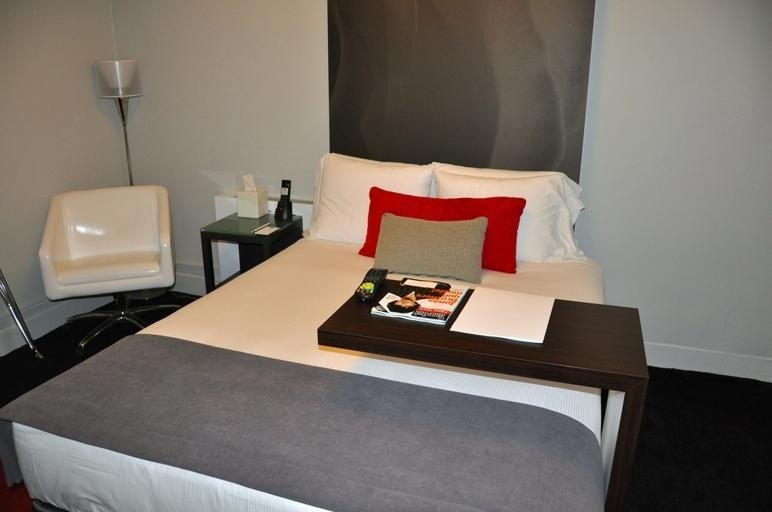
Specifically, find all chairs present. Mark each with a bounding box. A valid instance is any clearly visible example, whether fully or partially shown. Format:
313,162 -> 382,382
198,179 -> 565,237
37,183 -> 181,349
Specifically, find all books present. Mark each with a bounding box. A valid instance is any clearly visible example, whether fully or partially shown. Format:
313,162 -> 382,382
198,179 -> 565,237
371,277 -> 468,326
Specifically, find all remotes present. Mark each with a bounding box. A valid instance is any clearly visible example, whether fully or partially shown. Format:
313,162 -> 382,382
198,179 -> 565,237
355,268 -> 387,301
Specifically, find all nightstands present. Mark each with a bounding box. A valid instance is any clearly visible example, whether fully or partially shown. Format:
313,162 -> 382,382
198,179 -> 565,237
199,212 -> 303,295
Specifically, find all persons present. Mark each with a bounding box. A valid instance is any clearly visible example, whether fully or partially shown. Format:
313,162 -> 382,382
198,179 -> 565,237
386,277 -> 451,313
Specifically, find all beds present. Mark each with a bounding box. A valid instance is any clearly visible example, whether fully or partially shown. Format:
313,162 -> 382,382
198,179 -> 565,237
1,151 -> 652,511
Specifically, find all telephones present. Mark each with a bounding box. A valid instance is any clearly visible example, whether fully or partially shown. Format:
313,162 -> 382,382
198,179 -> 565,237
275,180 -> 292,226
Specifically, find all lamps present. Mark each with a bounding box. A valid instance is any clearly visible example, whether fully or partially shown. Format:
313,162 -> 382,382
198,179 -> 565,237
92,59 -> 142,187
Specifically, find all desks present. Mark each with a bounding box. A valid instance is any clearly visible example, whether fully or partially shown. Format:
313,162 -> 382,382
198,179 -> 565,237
317,267 -> 650,510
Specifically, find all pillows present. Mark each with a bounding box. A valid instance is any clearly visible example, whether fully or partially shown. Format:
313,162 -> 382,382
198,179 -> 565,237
373,212 -> 489,285
358,186 -> 526,274
301,154 -> 433,248
433,161 -> 585,264
